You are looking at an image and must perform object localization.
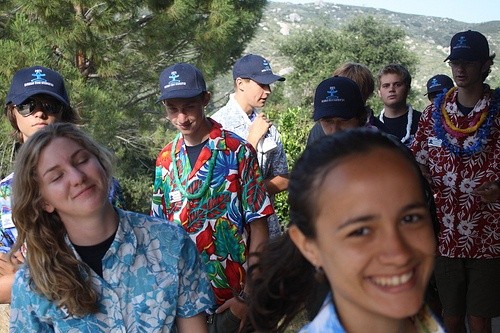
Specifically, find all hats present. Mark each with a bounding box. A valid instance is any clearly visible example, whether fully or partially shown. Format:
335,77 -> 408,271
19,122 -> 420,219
5,66 -> 69,106
424,74 -> 454,96
233,53 -> 285,85
313,75 -> 363,121
443,30 -> 489,63
154,63 -> 206,106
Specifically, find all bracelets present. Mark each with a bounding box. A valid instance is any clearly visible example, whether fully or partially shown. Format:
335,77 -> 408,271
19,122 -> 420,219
240,290 -> 249,299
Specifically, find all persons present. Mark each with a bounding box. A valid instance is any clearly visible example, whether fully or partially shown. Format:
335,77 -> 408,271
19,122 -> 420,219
242,128 -> 444,333
151,63 -> 275,333
305,64 -> 421,144
0,67 -> 126,304
10,124 -> 215,333
211,54 -> 289,269
410,30 -> 500,333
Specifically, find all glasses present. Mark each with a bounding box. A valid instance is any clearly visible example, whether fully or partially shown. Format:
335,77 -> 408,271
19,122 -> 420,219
448,60 -> 480,67
12,97 -> 63,116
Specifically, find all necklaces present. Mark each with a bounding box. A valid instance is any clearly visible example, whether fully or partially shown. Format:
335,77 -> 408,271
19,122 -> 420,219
172,134 -> 218,199
379,106 -> 412,142
441,83 -> 491,133
433,88 -> 500,153
